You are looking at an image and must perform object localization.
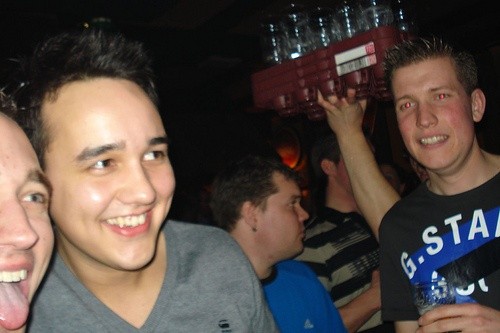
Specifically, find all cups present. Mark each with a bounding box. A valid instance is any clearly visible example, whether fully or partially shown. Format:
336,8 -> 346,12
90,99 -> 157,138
260,1 -> 414,64
414,279 -> 456,315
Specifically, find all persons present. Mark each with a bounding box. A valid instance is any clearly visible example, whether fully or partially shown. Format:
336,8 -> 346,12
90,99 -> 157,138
196,36 -> 500,333
25,29 -> 280,333
0,86 -> 55,333
